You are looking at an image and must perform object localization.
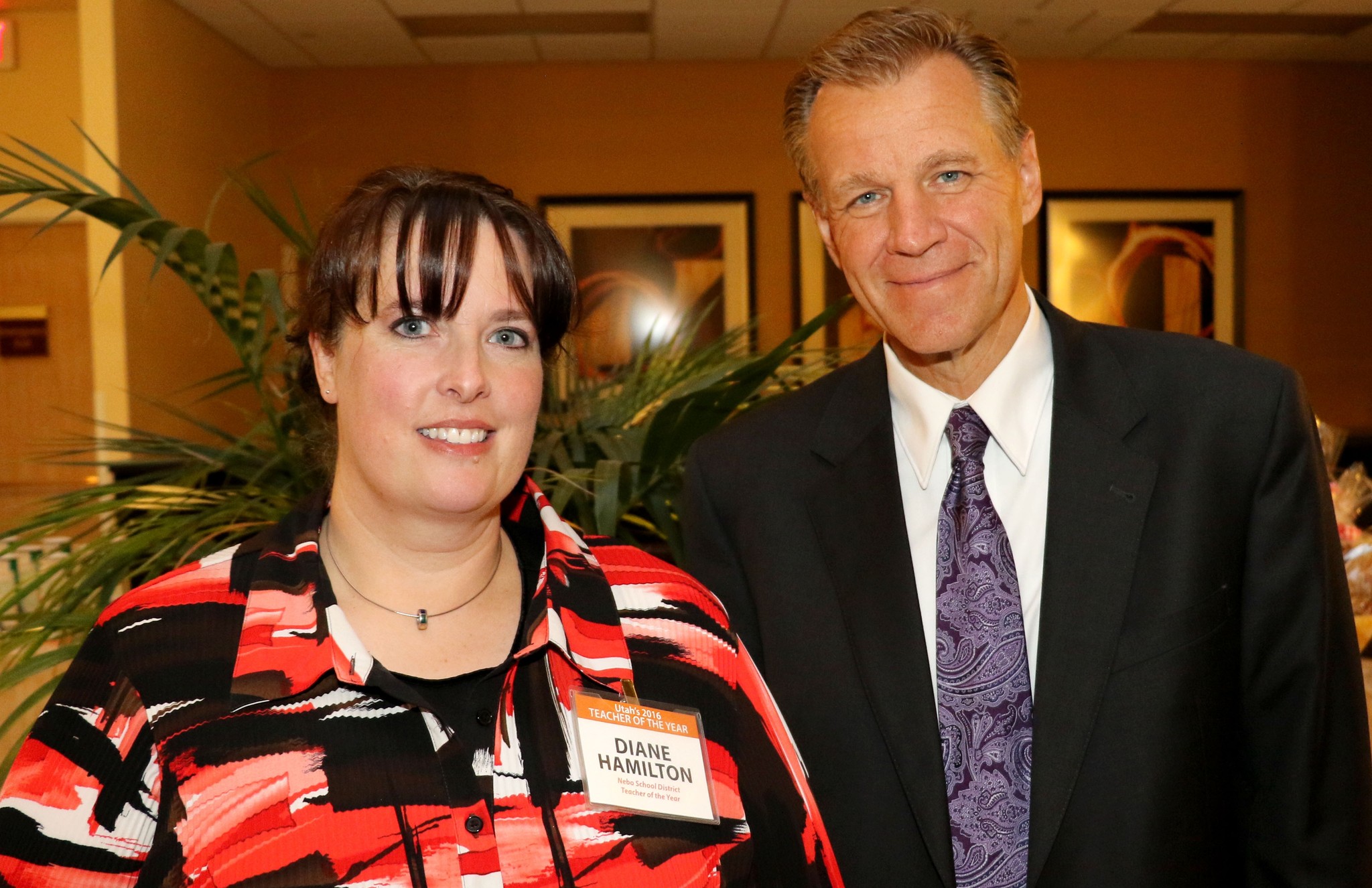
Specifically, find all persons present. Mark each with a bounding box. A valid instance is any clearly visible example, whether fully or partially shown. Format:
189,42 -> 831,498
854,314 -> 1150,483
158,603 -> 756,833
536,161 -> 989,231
1,162 -> 848,888
682,3 -> 1371,888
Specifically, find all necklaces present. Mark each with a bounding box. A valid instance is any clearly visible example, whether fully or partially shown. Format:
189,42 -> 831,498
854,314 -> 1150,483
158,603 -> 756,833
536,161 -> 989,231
325,513 -> 503,631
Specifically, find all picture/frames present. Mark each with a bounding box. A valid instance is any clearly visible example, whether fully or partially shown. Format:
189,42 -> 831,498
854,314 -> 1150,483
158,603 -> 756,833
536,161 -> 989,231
1037,190 -> 1249,352
535,189 -> 757,430
789,191 -> 886,390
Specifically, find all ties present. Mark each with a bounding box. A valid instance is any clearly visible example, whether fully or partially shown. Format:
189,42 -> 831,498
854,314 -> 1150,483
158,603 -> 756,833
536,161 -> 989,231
934,405 -> 1032,888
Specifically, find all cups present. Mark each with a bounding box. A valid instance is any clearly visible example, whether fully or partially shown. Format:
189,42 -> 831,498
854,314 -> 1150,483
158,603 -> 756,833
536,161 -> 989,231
0,536 -> 95,628
